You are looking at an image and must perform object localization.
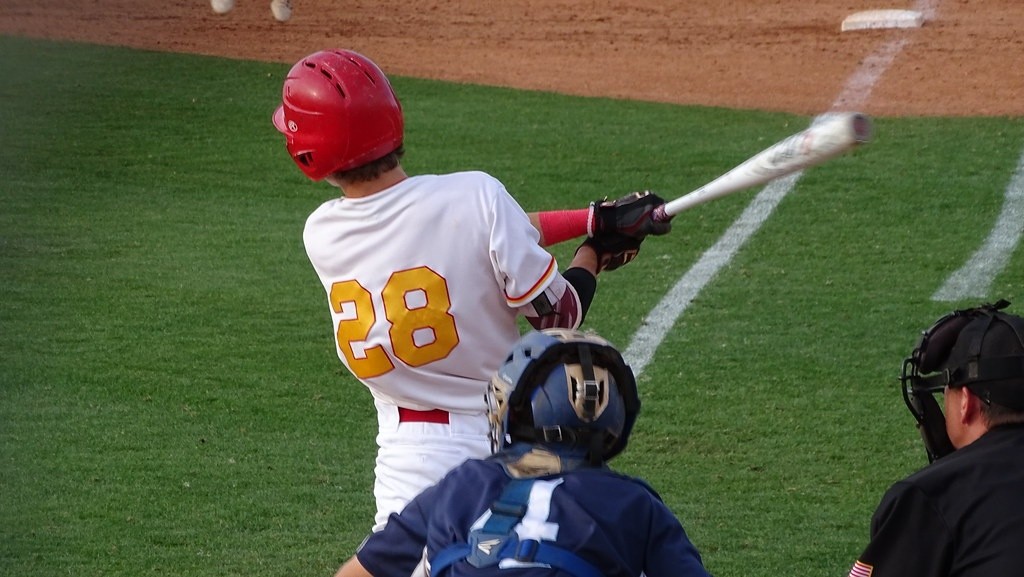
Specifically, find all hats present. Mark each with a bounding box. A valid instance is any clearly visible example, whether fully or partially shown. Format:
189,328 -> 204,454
944,307 -> 1024,406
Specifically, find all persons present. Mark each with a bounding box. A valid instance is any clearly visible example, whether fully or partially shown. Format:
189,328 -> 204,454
334,328 -> 707,577
847,297 -> 1024,576
270,50 -> 669,577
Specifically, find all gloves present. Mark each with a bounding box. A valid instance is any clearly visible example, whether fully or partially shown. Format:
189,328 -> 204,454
588,189 -> 672,242
574,232 -> 641,275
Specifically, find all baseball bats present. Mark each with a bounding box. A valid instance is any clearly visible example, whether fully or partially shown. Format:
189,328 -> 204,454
651,111 -> 873,225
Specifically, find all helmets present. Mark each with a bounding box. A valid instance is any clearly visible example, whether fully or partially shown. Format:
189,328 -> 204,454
272,49 -> 404,181
484,328 -> 641,460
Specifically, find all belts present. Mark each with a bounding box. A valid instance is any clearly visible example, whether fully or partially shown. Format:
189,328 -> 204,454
398,408 -> 449,424
